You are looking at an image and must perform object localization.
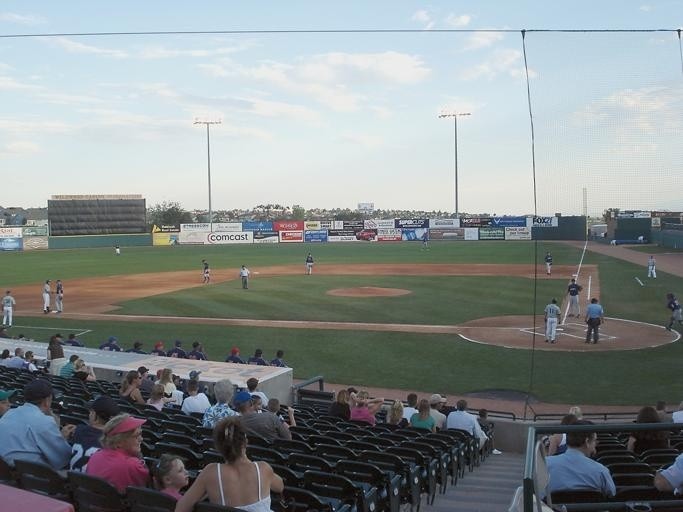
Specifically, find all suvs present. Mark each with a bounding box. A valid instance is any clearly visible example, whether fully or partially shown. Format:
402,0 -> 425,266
355,229 -> 375,241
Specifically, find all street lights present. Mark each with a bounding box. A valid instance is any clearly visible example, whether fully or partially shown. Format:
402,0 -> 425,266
436,112 -> 471,217
192,118 -> 222,222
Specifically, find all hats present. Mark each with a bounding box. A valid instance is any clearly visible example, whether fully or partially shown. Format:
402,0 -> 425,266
233,391 -> 255,403
429,392 -> 448,404
106,416 -> 145,434
25,379 -> 61,398
138,366 -> 150,374
108,335 -> 262,356
189,369 -> 201,377
88,394 -> 120,415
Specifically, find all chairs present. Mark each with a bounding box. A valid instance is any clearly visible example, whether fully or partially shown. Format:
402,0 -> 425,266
589,416 -> 681,501
0,365 -> 495,512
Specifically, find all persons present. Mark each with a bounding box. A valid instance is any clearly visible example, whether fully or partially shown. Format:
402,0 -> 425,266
306,253 -> 314,275
240,266 -> 250,289
648,255 -> 657,279
1,291 -> 16,328
423,236 -> 428,248
638,236 -> 644,244
328,388 -> 502,455
566,279 -> 583,319
544,297 -> 561,344
43,279 -> 52,313
55,279 -> 63,313
202,259 -> 210,284
585,298 -> 604,344
540,400 -> 683,495
611,240 -> 617,248
665,292 -> 683,331
545,252 -> 553,275
115,245 -> 120,257
